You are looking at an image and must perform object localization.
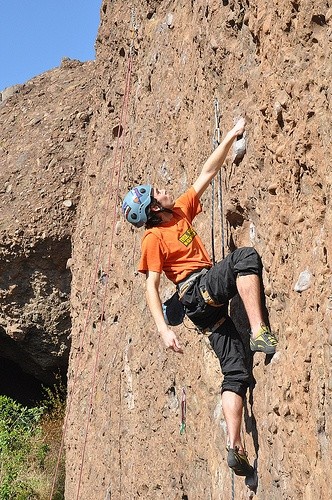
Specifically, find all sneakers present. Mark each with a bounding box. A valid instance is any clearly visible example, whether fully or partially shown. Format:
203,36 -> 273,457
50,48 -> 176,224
247,326 -> 279,354
225,445 -> 254,476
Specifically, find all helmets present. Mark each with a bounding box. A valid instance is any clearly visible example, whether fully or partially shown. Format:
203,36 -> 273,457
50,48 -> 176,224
121,184 -> 155,229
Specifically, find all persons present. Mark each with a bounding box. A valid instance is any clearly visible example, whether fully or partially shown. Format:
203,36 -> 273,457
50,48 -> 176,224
121,117 -> 278,477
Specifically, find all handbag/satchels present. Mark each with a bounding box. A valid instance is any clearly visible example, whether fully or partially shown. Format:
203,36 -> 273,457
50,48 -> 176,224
161,291 -> 186,326
